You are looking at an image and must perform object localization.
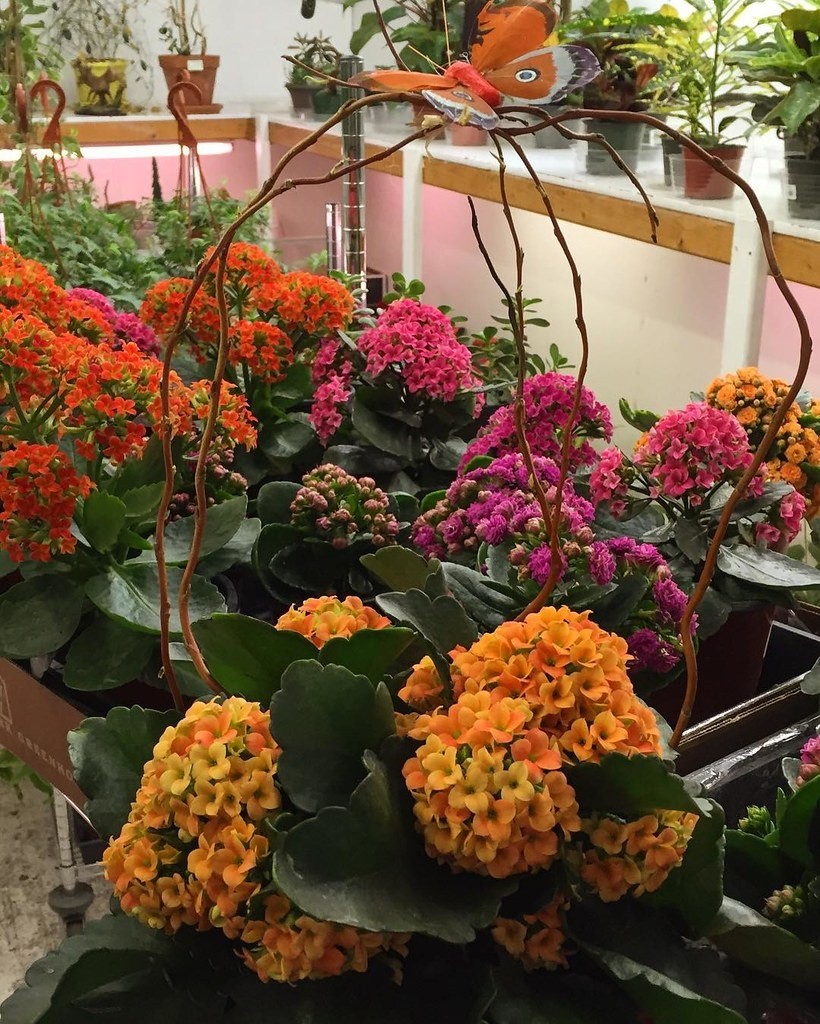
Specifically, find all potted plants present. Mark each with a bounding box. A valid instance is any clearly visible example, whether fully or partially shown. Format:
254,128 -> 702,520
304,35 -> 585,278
42,0 -> 152,115
283,0 -> 820,221
156,1 -> 221,106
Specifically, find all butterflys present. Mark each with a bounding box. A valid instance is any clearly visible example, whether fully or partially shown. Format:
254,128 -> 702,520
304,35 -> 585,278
346,1 -> 605,131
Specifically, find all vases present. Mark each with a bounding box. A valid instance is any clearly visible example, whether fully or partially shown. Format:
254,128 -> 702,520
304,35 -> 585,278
686,598 -> 779,720
0,654 -> 111,817
673,615 -> 820,776
683,713 -> 820,823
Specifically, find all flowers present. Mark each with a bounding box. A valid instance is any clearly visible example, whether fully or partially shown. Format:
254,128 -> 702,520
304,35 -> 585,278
0,131 -> 820,1024
